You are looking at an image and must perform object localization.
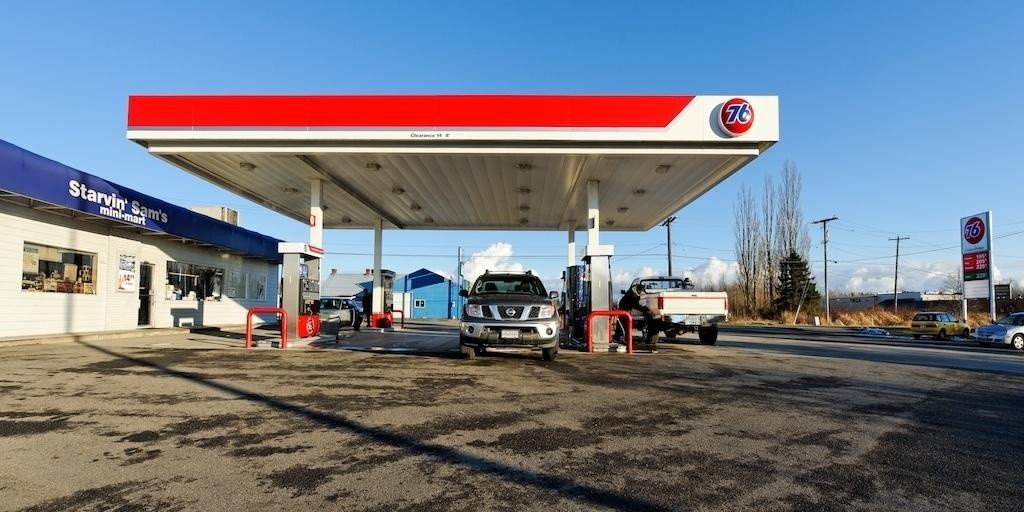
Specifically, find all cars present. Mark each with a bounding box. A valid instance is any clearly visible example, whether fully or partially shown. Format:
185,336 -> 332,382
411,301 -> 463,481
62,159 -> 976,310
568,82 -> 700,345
975,311 -> 1024,351
910,311 -> 971,340
318,298 -> 364,335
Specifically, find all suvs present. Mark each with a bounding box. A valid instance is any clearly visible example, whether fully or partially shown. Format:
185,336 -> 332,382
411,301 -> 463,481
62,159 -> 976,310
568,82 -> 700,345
459,269 -> 560,362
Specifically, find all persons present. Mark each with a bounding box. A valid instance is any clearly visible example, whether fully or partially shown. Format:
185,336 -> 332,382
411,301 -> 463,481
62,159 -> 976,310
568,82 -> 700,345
517,280 -> 531,293
612,283 -> 657,340
362,288 -> 371,328
324,299 -> 334,308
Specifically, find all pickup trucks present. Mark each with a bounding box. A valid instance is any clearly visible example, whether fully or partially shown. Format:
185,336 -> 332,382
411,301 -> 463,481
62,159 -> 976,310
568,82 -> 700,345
621,275 -> 731,347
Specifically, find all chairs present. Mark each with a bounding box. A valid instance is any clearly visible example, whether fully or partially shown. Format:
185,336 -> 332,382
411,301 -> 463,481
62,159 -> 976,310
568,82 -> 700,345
324,300 -> 331,308
484,283 -> 498,291
515,285 -> 530,292
